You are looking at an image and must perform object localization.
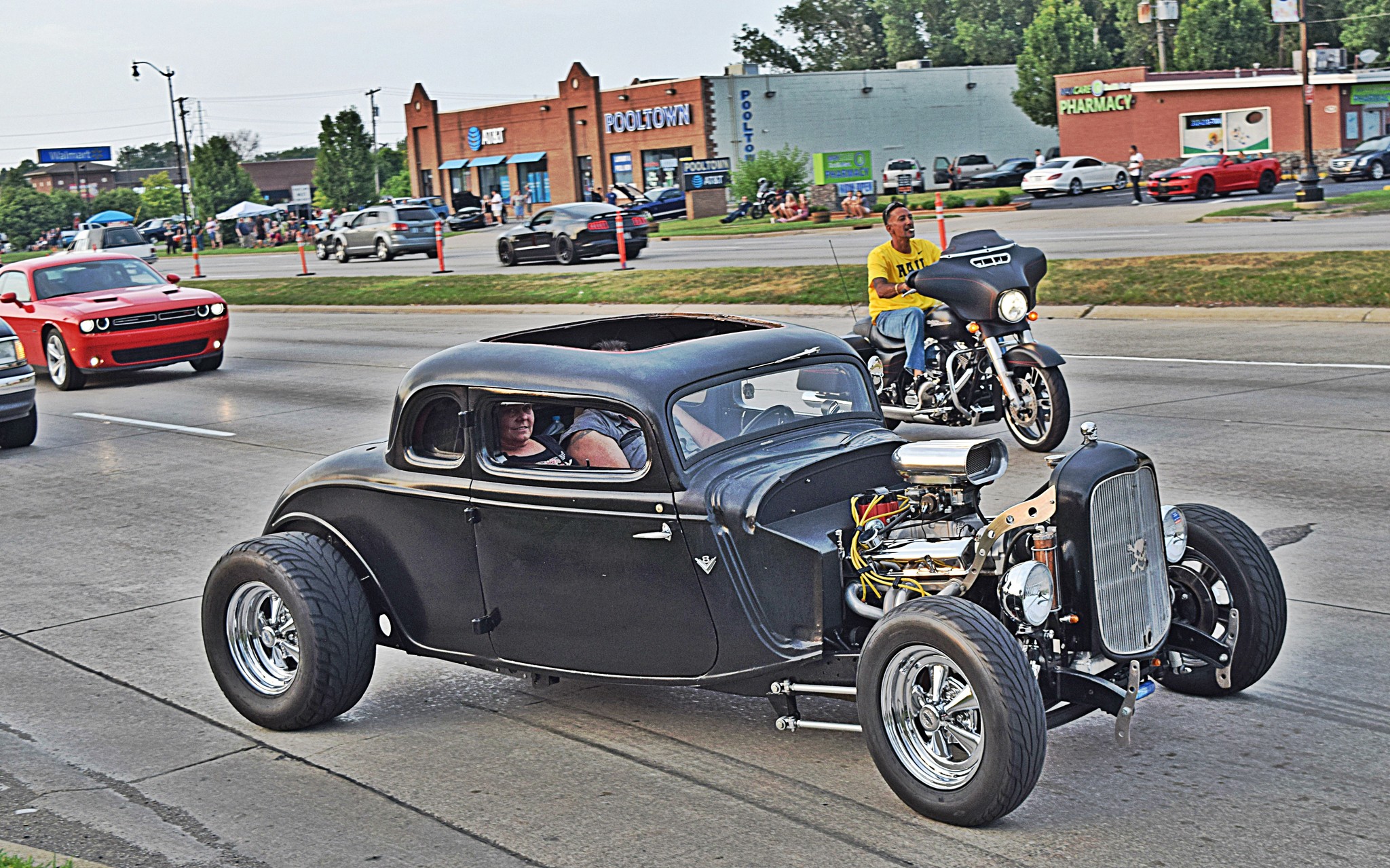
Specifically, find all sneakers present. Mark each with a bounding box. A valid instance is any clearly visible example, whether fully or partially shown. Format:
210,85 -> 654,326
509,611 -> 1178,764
914,374 -> 936,408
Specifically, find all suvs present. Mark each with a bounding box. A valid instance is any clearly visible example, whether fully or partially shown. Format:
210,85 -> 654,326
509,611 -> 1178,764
880,156 -> 926,195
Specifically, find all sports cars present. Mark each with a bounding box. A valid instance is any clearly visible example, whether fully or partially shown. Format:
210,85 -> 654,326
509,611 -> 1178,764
1147,154 -> 1281,202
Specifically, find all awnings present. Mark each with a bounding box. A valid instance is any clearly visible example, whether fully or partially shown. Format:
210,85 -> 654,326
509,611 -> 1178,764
437,159 -> 470,169
505,152 -> 546,164
467,155 -> 506,167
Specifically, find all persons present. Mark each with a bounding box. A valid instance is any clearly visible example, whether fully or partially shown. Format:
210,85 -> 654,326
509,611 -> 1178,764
720,195 -> 753,224
32,227 -> 62,251
1034,149 -> 1044,168
590,187 -> 602,203
768,193 -> 809,223
483,195 -> 493,213
494,401 -> 582,469
1238,151 -> 1246,159
234,206 -> 349,249
1219,148 -> 1224,155
605,186 -> 618,206
867,200 -> 947,408
164,217 -> 203,254
1127,145 -> 1144,204
487,190 -> 503,227
841,190 -> 870,219
204,216 -> 224,249
559,338 -> 727,468
511,185 -> 533,220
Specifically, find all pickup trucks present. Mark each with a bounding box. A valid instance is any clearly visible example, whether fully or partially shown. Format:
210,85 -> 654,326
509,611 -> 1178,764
932,154 -> 998,190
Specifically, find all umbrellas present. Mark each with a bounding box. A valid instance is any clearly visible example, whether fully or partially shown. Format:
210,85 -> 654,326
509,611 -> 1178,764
86,210 -> 134,224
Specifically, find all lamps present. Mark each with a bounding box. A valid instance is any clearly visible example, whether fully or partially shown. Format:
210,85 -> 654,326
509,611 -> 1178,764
862,87 -> 873,93
766,92 -> 776,98
618,94 -> 629,101
967,83 -> 976,89
576,120 -> 587,125
665,89 -> 676,95
540,105 -> 550,112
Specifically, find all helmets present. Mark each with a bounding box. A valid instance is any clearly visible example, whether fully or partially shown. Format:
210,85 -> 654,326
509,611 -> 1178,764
757,177 -> 766,185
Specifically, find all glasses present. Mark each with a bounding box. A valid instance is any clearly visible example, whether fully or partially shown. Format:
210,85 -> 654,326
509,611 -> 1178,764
885,201 -> 903,221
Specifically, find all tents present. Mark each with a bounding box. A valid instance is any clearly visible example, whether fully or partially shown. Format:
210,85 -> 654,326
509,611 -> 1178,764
215,201 -> 282,224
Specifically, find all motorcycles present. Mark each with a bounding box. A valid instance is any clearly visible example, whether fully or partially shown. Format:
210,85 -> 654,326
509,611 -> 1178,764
797,228 -> 1071,454
751,178 -> 800,220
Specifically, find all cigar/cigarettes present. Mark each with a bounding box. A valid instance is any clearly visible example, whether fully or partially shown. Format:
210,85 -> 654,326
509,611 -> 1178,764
909,228 -> 914,230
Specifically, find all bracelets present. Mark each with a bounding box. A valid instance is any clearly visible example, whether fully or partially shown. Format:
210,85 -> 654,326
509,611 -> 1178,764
895,284 -> 899,294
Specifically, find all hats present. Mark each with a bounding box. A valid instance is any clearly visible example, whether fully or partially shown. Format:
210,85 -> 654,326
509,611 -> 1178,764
522,185 -> 529,188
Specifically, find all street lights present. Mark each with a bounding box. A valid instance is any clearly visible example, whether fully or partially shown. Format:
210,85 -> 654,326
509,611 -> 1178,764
131,60 -> 193,252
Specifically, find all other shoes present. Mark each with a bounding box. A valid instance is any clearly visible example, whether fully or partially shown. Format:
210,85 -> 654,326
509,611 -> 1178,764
496,223 -> 503,227
1131,200 -> 1140,204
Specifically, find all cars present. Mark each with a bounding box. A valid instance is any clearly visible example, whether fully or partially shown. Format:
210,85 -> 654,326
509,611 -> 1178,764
1047,146 -> 1060,162
380,197 -> 450,220
56,223 -> 107,247
1021,157 -> 1130,197
311,212 -> 369,261
67,225 -> 159,266
447,190 -> 486,231
0,250 -> 230,391
1327,135 -> 1390,182
136,218 -> 186,245
614,183 -> 687,222
0,317 -> 38,449
495,202 -> 648,265
197,307 -> 1289,836
332,203 -> 446,263
968,159 -> 1038,190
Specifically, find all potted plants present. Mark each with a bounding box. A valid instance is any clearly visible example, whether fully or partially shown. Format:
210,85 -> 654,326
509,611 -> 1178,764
811,205 -> 831,223
